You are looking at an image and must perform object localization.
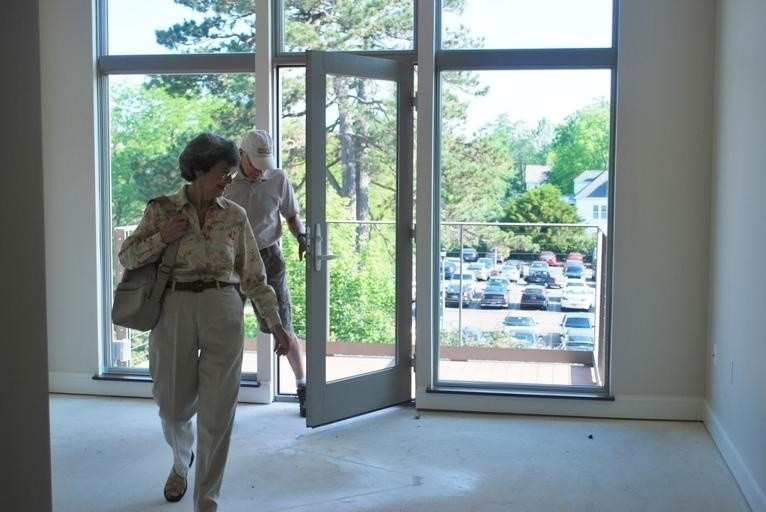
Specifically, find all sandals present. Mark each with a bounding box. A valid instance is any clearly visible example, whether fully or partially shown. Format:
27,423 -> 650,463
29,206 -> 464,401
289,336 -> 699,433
164,450 -> 194,503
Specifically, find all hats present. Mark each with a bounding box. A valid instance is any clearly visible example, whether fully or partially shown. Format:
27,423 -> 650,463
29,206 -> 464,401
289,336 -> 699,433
239,128 -> 276,171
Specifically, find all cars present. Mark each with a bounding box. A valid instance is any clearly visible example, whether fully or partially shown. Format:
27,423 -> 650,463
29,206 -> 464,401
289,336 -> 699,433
444,243 -> 598,347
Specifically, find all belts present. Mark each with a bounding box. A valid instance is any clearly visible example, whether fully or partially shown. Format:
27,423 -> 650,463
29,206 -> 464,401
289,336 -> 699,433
259,242 -> 280,256
168,280 -> 234,293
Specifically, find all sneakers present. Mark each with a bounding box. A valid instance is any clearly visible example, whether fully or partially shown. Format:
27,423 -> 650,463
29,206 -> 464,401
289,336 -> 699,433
298,382 -> 307,417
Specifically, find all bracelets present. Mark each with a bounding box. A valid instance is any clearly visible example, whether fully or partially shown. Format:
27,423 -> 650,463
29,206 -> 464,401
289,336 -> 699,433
296,233 -> 307,243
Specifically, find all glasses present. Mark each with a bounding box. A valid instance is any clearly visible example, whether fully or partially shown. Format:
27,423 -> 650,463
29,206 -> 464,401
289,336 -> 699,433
208,168 -> 236,177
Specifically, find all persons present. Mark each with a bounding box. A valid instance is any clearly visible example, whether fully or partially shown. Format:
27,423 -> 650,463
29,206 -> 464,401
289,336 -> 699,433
219,129 -> 309,418
118,132 -> 290,512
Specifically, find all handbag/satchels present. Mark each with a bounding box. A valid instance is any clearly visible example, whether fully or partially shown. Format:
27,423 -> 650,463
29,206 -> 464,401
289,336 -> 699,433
111,194 -> 181,332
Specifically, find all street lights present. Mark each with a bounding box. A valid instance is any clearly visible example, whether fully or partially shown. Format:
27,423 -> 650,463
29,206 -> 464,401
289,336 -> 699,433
438,246 -> 448,319
